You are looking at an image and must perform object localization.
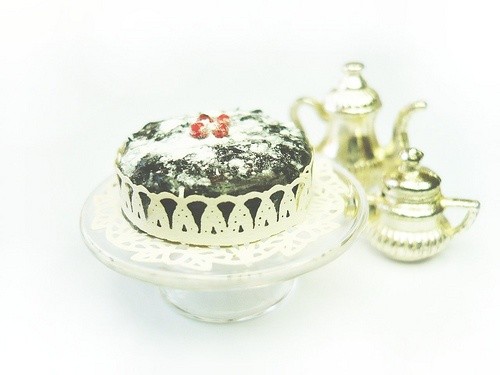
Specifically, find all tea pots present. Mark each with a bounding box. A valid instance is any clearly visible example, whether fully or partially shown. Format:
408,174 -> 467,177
290,59 -> 428,218
347,147 -> 481,267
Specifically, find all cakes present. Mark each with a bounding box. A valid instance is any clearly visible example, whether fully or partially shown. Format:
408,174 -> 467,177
113,105 -> 314,250
